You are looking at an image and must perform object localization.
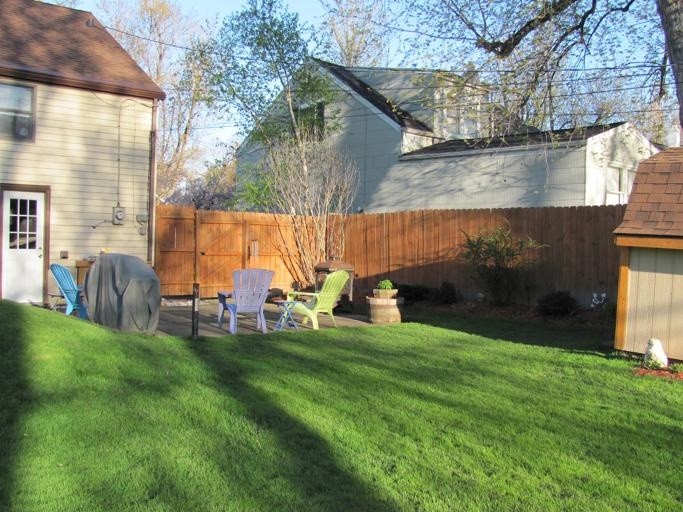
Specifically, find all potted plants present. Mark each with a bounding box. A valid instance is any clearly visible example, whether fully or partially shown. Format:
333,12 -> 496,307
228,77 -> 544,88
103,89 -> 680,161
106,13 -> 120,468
372,278 -> 399,299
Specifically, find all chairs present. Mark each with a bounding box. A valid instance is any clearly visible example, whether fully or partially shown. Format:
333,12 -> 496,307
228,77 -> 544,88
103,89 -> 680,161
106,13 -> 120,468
282,269 -> 349,331
217,267 -> 274,335
50,262 -> 88,321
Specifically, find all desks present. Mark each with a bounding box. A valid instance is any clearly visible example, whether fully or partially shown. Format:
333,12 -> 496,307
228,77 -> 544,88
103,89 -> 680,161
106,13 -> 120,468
271,299 -> 301,333
